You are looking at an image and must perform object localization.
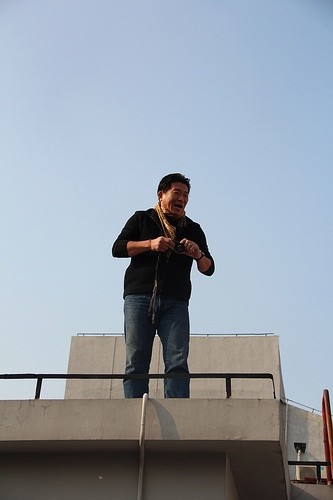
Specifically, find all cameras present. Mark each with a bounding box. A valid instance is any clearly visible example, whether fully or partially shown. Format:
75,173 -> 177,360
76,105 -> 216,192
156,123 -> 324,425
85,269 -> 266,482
167,240 -> 188,252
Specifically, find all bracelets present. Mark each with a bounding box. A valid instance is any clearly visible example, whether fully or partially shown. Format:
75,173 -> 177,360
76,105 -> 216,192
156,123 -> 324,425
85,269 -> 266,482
149,239 -> 152,252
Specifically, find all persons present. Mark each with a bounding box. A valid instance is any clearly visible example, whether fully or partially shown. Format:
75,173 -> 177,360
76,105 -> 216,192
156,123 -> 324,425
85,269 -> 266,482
111,172 -> 216,399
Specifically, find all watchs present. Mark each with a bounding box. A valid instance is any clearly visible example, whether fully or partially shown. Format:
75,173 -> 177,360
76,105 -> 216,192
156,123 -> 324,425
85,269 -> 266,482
195,252 -> 205,262
